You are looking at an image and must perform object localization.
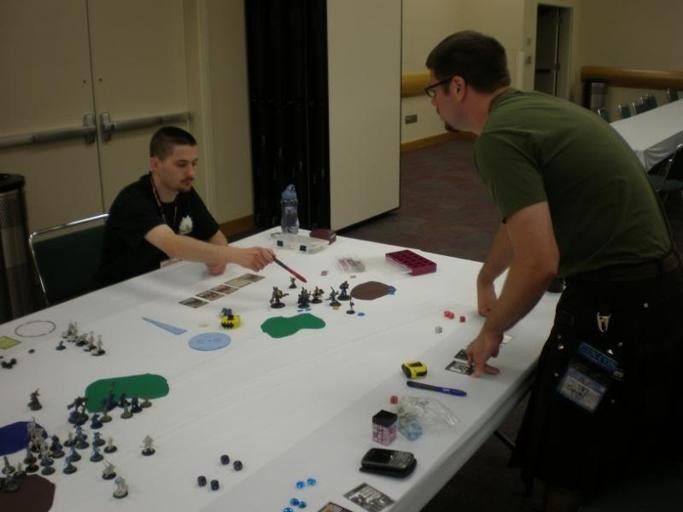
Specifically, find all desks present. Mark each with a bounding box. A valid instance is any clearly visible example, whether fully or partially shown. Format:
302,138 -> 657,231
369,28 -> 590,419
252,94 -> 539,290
0,223 -> 565,512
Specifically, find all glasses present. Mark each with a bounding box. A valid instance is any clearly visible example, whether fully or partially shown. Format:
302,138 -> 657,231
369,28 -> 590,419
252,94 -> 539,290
423,78 -> 451,99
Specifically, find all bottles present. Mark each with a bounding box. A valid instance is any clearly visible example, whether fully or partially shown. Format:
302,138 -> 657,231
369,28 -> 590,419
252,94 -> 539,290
280,184 -> 300,235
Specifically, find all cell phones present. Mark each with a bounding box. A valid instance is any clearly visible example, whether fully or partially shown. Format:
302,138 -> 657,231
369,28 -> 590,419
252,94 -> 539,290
358,447 -> 417,478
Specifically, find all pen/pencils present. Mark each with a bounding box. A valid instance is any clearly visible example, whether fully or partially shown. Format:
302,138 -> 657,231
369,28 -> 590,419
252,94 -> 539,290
272,256 -> 307,283
407,381 -> 466,396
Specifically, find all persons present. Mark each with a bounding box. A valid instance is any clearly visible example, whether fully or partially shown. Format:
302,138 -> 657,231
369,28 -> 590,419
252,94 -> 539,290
422,31 -> 682,512
81,123 -> 276,284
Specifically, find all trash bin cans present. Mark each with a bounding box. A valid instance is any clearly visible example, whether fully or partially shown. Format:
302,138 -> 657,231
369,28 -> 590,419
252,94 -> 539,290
0,174 -> 35,325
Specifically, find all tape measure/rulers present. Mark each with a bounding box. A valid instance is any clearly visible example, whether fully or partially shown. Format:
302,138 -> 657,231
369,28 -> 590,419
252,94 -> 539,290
221,315 -> 241,329
402,361 -> 427,379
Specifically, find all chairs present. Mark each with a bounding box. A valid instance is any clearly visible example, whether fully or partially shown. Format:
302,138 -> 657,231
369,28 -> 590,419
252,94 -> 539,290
28,211 -> 114,308
596,88 -> 683,193
